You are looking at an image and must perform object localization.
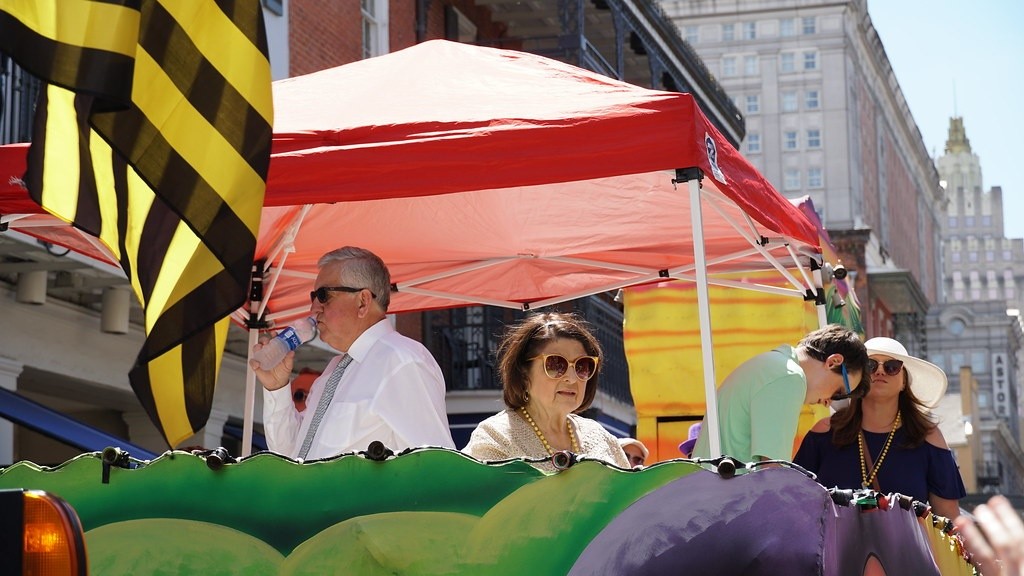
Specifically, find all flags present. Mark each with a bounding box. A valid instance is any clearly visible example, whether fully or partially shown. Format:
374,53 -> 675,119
0,1 -> 275,450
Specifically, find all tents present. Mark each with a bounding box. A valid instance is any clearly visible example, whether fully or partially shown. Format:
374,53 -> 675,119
0,40 -> 832,469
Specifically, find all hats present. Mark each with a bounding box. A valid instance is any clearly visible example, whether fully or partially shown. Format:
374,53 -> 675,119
617,438 -> 649,458
679,423 -> 702,455
863,337 -> 948,413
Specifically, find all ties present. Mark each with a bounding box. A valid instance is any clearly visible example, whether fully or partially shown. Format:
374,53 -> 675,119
297,353 -> 352,459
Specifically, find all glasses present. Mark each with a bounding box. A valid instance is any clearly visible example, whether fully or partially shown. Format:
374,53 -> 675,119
867,359 -> 903,376
832,362 -> 852,401
527,353 -> 599,381
310,286 -> 377,303
625,452 -> 644,464
687,452 -> 692,459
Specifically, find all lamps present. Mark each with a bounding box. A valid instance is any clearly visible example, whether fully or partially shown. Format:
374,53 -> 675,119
83,286 -> 130,335
15,270 -> 48,305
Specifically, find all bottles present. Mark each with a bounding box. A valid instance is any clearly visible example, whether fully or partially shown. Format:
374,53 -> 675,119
252,315 -> 317,370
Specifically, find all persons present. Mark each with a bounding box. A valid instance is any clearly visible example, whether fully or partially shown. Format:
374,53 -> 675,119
679,422 -> 703,459
461,311 -> 632,468
618,438 -> 649,467
793,336 -> 966,518
251,246 -> 457,462
690,325 -> 870,472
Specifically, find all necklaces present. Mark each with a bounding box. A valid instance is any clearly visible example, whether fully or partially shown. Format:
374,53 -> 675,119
859,411 -> 901,486
520,406 -> 581,454
